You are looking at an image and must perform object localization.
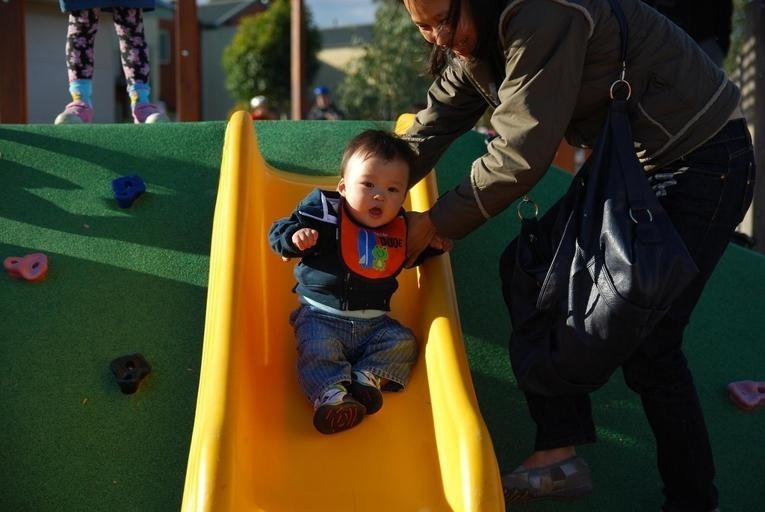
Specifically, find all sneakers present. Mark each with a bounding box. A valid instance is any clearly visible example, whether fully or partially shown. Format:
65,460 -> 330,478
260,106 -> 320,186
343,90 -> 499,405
500,449 -> 599,504
312,368 -> 384,436
54,101 -> 94,124
132,101 -> 165,123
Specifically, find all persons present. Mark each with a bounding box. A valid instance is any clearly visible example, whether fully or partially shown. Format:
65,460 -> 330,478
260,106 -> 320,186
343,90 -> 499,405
53,0 -> 172,124
400,0 -> 755,512
250,86 -> 271,120
267,129 -> 453,434
306,85 -> 345,120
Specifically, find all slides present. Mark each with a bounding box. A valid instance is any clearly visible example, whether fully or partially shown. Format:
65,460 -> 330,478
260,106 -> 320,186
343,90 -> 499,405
181,110 -> 506,512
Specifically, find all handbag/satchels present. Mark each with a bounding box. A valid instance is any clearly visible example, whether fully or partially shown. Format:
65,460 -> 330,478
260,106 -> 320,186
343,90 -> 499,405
497,97 -> 698,398
